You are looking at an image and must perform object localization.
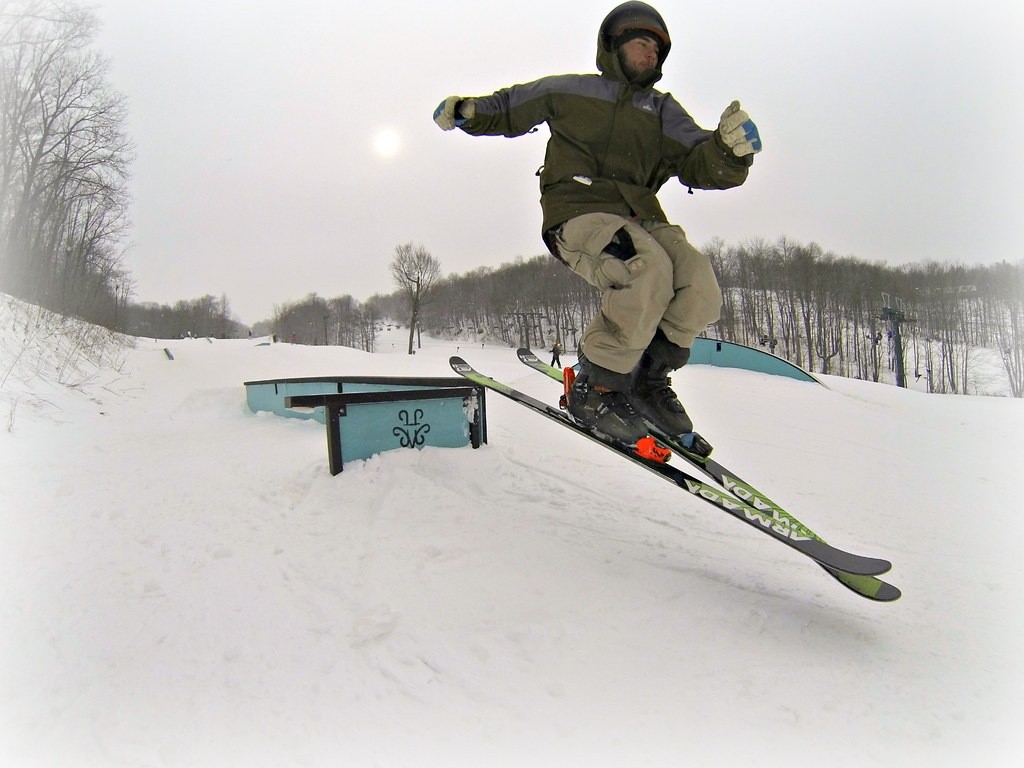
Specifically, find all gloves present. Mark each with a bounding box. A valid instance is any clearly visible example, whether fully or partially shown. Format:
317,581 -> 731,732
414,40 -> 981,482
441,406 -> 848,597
719,100 -> 763,157
432,96 -> 475,131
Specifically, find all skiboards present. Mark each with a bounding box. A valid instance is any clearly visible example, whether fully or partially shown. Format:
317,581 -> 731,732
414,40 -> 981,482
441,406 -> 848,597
447,344 -> 903,604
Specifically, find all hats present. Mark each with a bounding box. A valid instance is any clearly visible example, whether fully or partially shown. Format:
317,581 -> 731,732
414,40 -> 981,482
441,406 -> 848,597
607,11 -> 670,49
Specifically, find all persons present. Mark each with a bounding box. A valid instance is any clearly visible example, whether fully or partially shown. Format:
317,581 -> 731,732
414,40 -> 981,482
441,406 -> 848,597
248,330 -> 252,340
291,332 -> 297,345
433,1 -> 762,443
549,344 -> 562,368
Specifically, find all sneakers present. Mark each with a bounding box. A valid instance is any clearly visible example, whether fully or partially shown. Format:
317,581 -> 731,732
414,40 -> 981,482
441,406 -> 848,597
569,374 -> 648,442
632,377 -> 694,434
